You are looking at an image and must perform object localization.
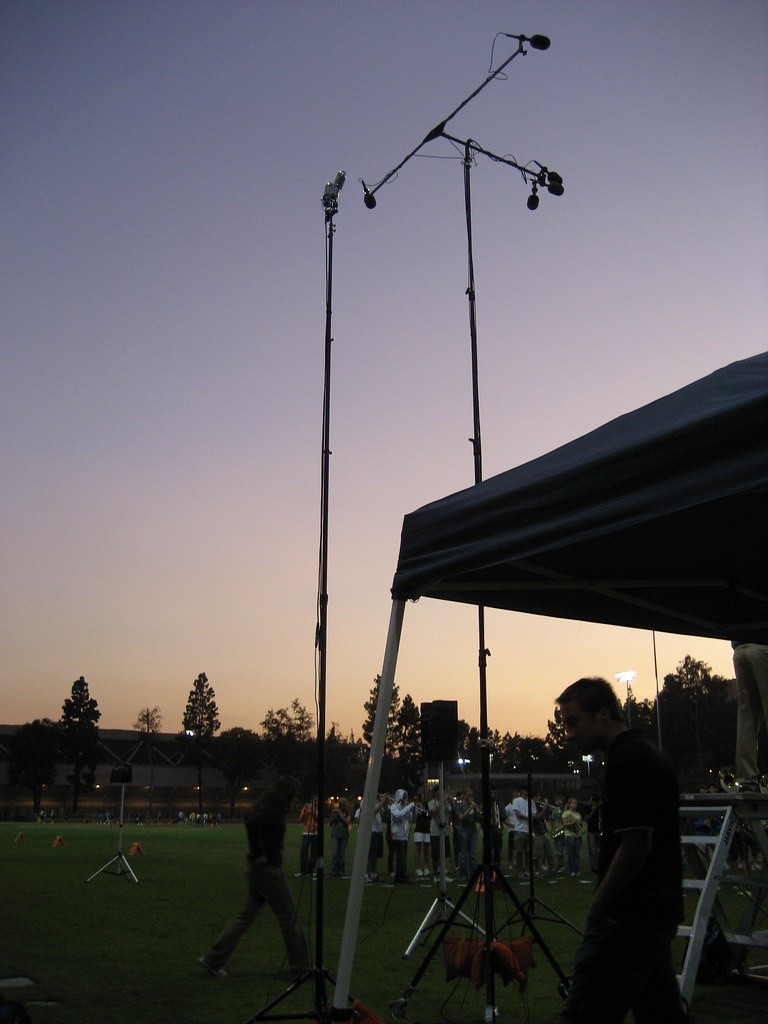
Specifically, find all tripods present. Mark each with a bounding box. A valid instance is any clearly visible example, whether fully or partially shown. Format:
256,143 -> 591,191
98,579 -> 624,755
404,758 -> 496,959
369,44 -> 573,1017
86,784 -> 139,883
491,773 -> 589,943
249,203 -> 386,1024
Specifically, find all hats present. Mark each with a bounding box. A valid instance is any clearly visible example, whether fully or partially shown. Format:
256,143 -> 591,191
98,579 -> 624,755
522,783 -> 532,790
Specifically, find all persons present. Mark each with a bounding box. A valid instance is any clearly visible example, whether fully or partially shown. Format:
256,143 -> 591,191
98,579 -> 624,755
555,675 -> 686,1024
199,740 -> 315,982
294,780 -> 768,884
34,807 -> 225,827
728,640 -> 768,793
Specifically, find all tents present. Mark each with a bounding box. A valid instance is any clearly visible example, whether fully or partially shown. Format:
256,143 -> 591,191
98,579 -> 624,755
332,351 -> 767,1013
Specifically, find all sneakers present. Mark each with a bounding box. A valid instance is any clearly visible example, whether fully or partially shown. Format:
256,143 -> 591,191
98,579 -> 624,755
433,875 -> 453,883
365,875 -> 372,883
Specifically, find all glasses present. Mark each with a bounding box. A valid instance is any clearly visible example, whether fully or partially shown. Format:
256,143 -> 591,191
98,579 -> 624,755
564,713 -> 590,729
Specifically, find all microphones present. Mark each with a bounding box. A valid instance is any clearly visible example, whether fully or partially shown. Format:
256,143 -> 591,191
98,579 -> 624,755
361,179 -> 376,209
330,170 -> 348,208
506,34 -> 550,50
322,182 -> 332,206
527,161 -> 564,210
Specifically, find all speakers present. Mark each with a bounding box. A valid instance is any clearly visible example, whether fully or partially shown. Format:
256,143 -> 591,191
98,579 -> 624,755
111,763 -> 133,783
419,700 -> 458,762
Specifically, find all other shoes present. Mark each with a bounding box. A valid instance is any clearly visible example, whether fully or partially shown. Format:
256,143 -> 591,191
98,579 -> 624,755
312,873 -> 317,877
294,873 -> 302,878
571,872 -> 581,877
739,782 -> 761,793
521,873 -> 531,879
724,864 -> 761,870
542,866 -> 565,874
391,872 -> 396,876
332,872 -> 345,876
199,957 -> 228,977
416,869 -> 431,876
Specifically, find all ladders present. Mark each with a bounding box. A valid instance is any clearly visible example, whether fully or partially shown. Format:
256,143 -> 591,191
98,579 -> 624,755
672,804 -> 768,1015
